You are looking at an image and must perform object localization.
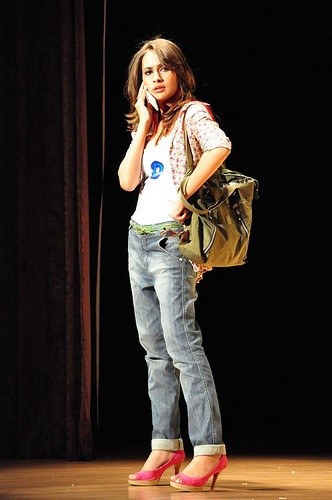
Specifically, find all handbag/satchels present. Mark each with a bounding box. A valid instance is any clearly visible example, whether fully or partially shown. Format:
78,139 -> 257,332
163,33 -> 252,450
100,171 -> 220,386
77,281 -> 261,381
179,168 -> 260,269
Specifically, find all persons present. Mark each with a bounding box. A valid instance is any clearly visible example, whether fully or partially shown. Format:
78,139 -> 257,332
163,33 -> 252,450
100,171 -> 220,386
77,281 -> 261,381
115,36 -> 231,493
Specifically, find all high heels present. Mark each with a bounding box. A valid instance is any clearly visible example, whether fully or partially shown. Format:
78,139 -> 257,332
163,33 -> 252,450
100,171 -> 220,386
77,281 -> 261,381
170,454 -> 228,492
128,449 -> 184,486
